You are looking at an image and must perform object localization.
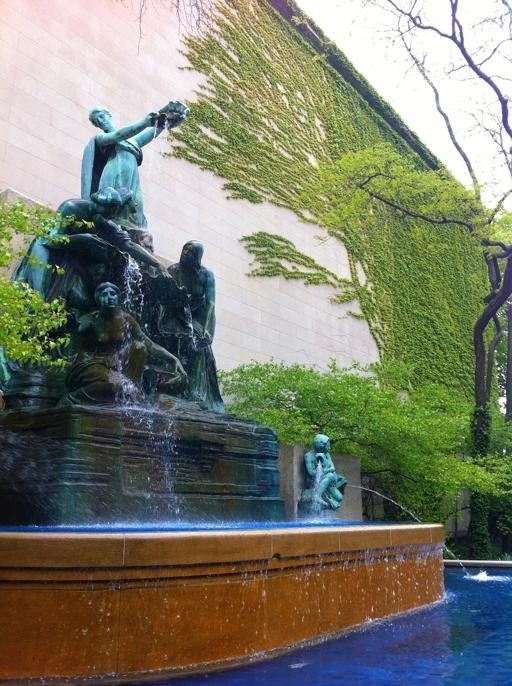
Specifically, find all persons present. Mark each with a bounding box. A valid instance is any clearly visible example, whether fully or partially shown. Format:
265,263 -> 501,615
304,434 -> 346,517
80,99 -> 190,256
14,188 -> 217,407
